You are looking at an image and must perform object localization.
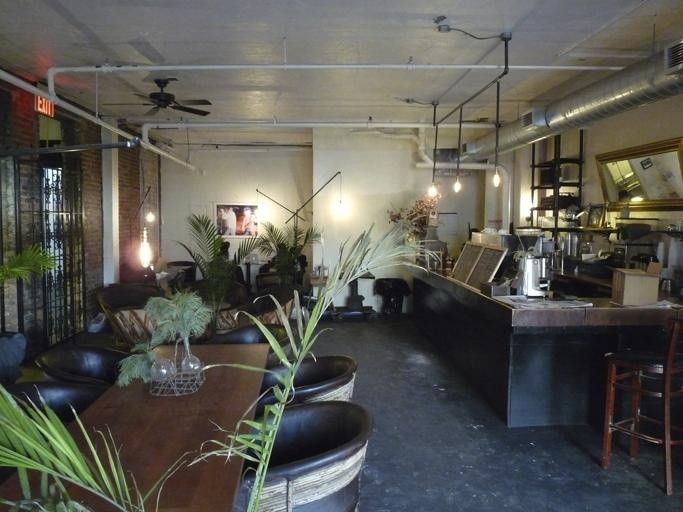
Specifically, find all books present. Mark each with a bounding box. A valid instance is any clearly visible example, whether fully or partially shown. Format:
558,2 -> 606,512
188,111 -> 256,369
534,300 -> 593,309
491,293 -> 546,310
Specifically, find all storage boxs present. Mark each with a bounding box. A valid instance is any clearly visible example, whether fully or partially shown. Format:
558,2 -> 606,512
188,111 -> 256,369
609,267 -> 660,307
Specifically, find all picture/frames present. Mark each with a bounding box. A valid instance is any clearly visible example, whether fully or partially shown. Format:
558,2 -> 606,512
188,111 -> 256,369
212,201 -> 264,239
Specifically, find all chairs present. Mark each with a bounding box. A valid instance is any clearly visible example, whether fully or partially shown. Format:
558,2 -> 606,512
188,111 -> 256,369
95,261 -> 301,347
600,321 -> 683,496
0,324 -> 374,511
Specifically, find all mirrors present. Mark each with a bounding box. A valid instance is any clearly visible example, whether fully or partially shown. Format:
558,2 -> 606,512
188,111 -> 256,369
593,137 -> 683,212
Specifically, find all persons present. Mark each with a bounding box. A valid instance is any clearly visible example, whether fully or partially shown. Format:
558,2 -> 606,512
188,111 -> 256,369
240,206 -> 256,235
220,206 -> 237,235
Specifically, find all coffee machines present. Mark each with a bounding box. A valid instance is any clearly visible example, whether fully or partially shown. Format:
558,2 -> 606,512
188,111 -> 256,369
516,251 -> 548,299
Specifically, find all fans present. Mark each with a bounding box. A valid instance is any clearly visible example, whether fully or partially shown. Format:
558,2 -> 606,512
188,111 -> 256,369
101,78 -> 212,117
